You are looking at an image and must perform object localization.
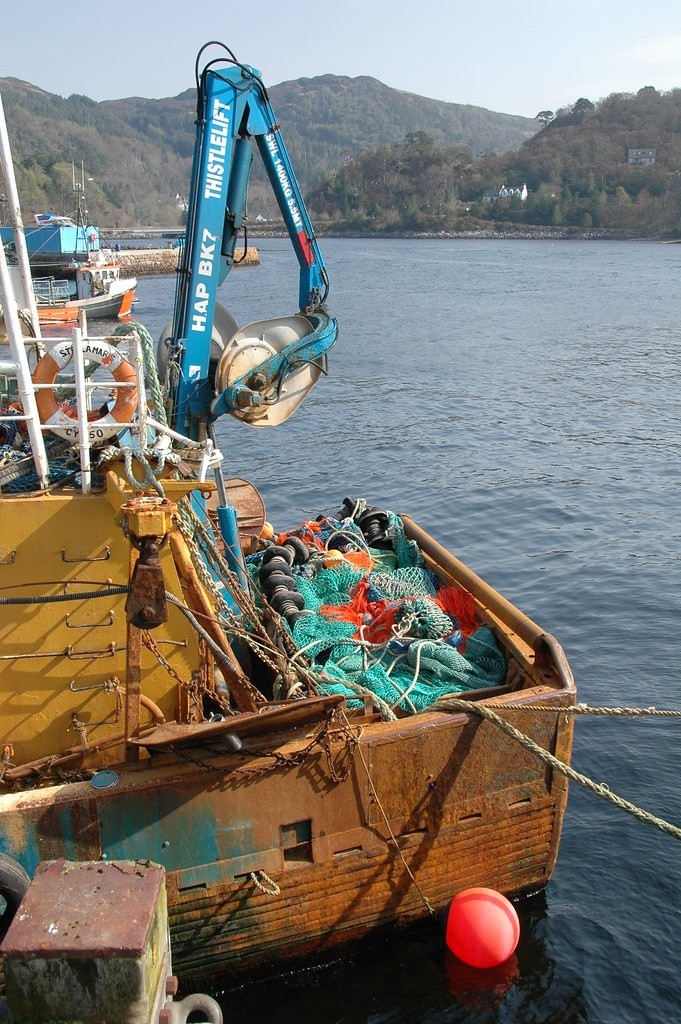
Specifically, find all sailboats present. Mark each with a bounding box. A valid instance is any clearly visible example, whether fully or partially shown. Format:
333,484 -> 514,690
0,159 -> 139,328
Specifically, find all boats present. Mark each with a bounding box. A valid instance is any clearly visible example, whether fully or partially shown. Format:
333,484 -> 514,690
0,39 -> 580,1023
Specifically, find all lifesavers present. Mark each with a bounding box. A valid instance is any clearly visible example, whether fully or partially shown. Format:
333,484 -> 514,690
33,340 -> 141,447
